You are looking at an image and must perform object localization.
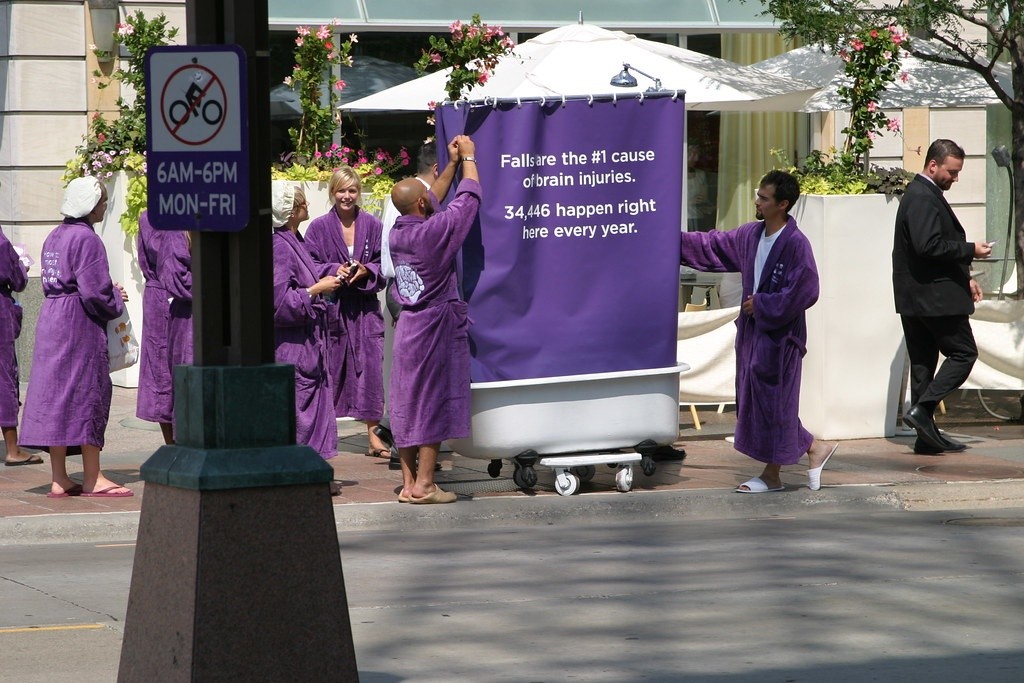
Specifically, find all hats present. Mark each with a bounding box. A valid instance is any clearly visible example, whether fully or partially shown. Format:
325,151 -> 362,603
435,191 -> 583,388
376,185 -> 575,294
60,175 -> 101,219
272,180 -> 294,228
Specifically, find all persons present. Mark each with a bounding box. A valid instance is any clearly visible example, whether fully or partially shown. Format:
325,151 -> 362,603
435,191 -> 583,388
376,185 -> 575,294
304,167 -> 392,458
893,140 -> 993,454
680,169 -> 839,493
0,225 -> 44,466
390,134 -> 482,504
271,179 -> 343,494
15,174 -> 135,497
372,142 -> 442,470
136,209 -> 193,445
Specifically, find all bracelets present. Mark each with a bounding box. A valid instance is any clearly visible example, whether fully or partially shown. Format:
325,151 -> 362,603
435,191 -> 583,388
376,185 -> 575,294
461,157 -> 477,164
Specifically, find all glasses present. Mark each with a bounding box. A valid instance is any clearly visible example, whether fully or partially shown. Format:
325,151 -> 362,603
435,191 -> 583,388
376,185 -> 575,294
294,201 -> 310,208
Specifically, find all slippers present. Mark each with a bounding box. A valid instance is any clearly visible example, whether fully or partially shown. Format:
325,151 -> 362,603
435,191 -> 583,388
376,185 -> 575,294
806,443 -> 839,490
365,449 -> 392,459
7,456 -> 43,465
409,483 -> 457,503
735,476 -> 787,494
47,484 -> 83,497
397,488 -> 414,502
80,487 -> 134,497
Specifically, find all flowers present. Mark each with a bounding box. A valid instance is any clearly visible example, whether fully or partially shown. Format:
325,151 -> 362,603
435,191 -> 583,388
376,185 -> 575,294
754,13 -> 919,196
51,4 -> 525,237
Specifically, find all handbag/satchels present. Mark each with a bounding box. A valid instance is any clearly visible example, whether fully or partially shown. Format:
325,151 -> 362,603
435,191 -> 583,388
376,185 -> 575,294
106,292 -> 140,373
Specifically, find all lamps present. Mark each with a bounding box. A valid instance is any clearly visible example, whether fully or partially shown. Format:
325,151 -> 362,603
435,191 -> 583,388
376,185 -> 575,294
610,62 -> 662,91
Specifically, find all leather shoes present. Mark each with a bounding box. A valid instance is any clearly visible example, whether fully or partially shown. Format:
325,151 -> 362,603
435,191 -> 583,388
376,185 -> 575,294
903,404 -> 948,449
914,436 -> 965,454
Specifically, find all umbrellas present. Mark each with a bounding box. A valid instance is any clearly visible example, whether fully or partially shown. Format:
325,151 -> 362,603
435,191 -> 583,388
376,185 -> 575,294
336,11 -> 822,110
707,29 -> 1014,112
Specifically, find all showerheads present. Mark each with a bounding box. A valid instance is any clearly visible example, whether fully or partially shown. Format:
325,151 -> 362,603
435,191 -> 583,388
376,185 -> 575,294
991,144 -> 1012,168
609,63 -> 662,89
